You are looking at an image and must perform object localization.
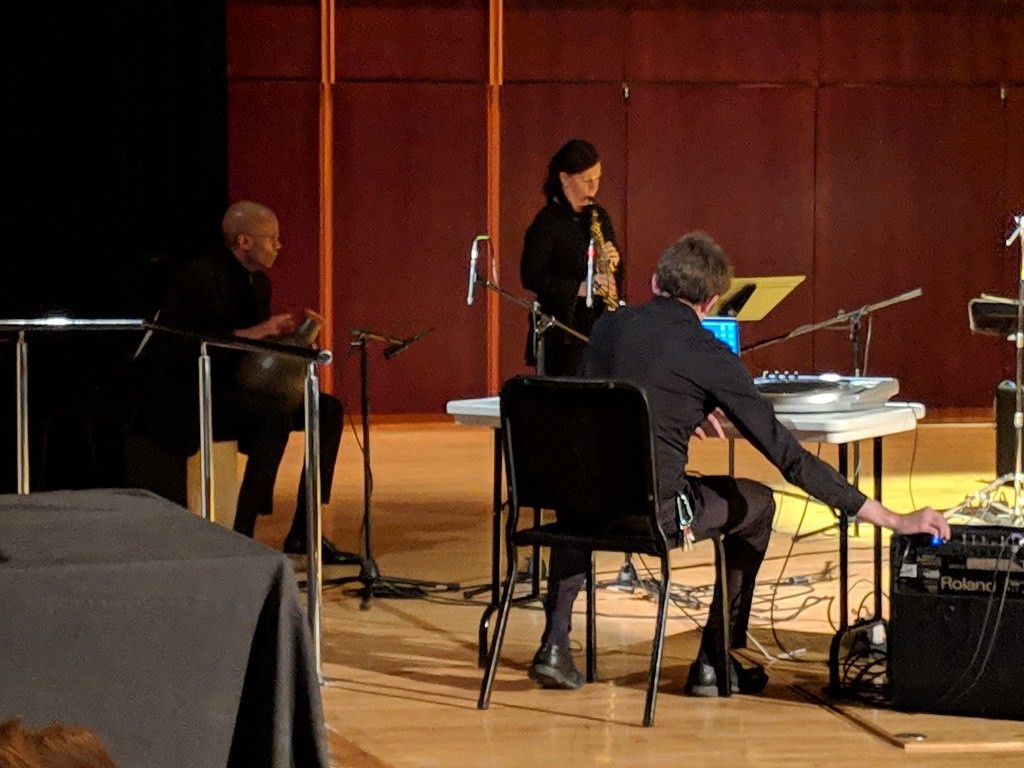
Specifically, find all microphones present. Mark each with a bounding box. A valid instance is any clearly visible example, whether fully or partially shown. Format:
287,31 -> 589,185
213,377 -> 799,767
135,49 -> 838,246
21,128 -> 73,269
586,239 -> 595,307
467,239 -> 478,304
382,326 -> 435,360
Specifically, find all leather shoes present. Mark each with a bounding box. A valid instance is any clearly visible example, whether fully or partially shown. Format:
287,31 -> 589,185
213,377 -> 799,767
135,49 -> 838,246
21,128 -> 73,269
283,530 -> 360,566
683,662 -> 769,698
526,643 -> 586,690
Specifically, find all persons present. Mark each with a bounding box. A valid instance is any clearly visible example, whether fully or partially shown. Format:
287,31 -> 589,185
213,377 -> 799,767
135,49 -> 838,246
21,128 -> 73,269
131,199 -> 363,565
527,231 -> 951,700
518,139 -> 624,377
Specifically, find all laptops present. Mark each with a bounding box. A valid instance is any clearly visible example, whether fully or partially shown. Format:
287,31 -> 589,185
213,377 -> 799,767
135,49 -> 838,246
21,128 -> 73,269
701,317 -> 741,358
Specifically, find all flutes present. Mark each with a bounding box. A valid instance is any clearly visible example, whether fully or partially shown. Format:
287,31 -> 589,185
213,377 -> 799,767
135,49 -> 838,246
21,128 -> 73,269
588,195 -> 619,313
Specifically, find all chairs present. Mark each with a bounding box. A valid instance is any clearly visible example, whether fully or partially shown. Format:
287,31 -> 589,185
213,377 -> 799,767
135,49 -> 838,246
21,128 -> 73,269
476,373 -> 732,728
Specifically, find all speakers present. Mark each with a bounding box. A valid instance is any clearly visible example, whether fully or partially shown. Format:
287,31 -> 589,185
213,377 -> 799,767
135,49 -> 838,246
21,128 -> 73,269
889,522 -> 1024,721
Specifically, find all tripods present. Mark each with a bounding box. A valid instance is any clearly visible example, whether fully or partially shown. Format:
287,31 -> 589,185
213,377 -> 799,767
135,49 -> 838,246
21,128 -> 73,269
463,277 -> 702,611
297,327 -> 462,612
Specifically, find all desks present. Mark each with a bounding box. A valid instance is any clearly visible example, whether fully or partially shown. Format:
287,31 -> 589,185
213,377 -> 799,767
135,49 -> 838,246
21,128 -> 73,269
0,487 -> 332,768
446,396 -> 926,698
